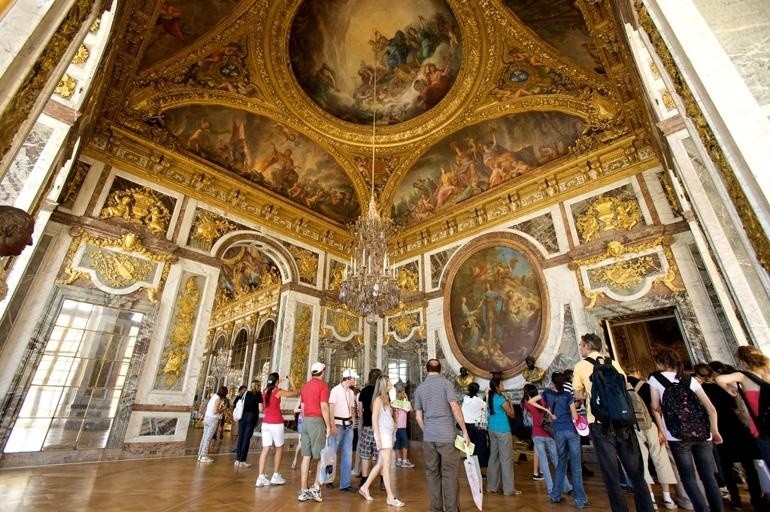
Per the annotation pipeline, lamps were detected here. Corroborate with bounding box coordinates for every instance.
[337,28,401,317]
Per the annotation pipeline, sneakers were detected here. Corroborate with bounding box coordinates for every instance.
[308,484,323,502]
[197,456,201,461]
[297,490,313,501]
[653,500,658,511]
[239,462,251,467]
[401,461,415,468]
[255,476,271,486]
[395,459,403,466]
[664,498,678,509]
[539,471,545,478]
[533,475,544,481]
[271,475,287,485]
[582,500,593,508]
[200,456,214,463]
[234,460,239,466]
[552,496,566,504]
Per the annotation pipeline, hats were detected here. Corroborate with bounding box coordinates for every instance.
[342,368,360,379]
[572,415,591,437]
[311,362,325,375]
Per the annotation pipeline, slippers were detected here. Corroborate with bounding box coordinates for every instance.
[291,463,297,470]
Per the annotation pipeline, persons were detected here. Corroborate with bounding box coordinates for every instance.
[191,344,770,512]
[570,332,657,512]
[452,281,542,373]
[0,205,35,258]
[328,268,345,291]
[607,240,625,258]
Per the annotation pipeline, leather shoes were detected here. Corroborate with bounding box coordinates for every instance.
[387,497,405,507]
[340,485,357,494]
[325,483,335,488]
[359,487,374,501]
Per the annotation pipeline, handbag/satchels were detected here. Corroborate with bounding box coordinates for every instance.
[541,418,554,436]
[318,435,337,485]
[736,369,770,439]
[464,443,484,511]
[474,393,489,431]
[233,391,248,421]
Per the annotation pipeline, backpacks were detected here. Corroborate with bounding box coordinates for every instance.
[584,355,641,432]
[631,379,653,430]
[649,370,711,441]
